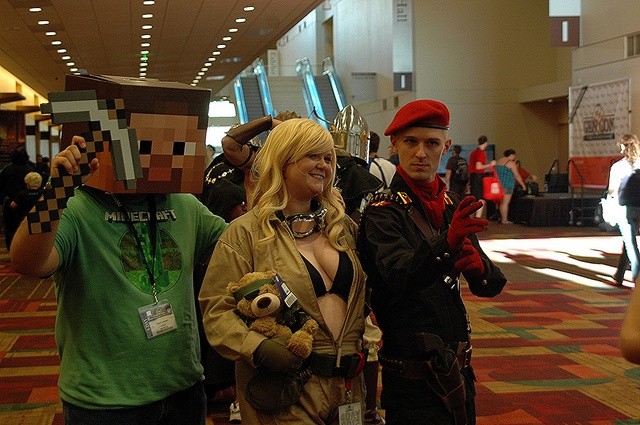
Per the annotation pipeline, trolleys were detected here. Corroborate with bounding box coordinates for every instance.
[567,159,598,227]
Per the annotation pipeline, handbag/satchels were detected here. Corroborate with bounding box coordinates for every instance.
[524,182,538,195]
[544,159,569,193]
[482,164,504,201]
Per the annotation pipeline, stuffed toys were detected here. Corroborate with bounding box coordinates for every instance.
[226,268,319,358]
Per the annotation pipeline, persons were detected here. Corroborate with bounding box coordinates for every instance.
[445,145,468,193]
[494,149,526,226]
[199,176,247,420]
[197,118,369,425]
[0,146,35,220]
[9,73,229,425]
[206,144,215,161]
[468,135,496,201]
[221,111,303,213]
[618,145,640,247]
[35,154,49,173]
[514,159,537,186]
[368,130,397,187]
[599,133,640,288]
[356,98,510,425]
[10,171,42,214]
[619,269,640,365]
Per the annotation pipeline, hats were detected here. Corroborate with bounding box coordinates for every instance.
[384,99,450,136]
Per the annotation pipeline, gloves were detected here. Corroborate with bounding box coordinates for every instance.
[454,238,484,276]
[254,339,302,395]
[447,196,489,258]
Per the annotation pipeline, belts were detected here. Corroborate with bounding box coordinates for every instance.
[302,349,369,378]
[375,346,473,376]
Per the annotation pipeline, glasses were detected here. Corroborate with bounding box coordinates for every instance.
[275,198,329,240]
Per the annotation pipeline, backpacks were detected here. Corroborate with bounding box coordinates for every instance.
[451,156,468,186]
